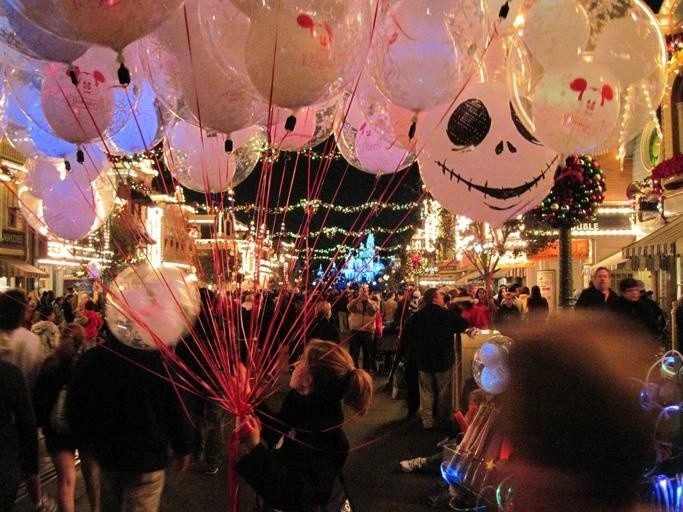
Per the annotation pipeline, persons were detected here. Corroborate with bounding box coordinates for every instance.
[2,266,683,511]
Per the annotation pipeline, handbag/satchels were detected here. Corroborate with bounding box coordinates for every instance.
[51,389,69,431]
[392,366,408,400]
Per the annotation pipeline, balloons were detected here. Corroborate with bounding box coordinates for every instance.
[1,2,667,349]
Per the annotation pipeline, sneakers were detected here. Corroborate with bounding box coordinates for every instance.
[450,497,485,511]
[399,457,422,472]
[427,493,449,507]
[32,498,57,512]
